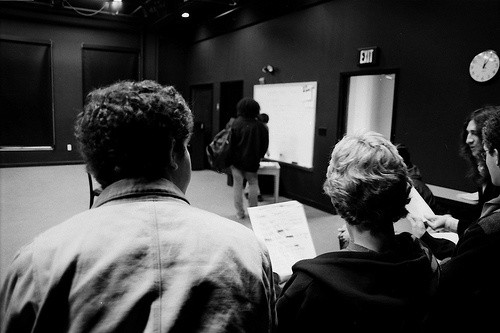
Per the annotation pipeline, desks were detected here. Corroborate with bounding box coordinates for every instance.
[256,161,280,202]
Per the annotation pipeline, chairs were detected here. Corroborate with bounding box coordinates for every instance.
[87,164,102,208]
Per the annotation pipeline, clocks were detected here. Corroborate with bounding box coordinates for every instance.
[469,50,500,83]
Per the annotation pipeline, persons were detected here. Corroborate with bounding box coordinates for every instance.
[0,80,276,333]
[273,131,440,333]
[226,97,268,218]
[397,105,500,333]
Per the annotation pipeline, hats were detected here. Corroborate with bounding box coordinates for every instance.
[238,97,260,118]
